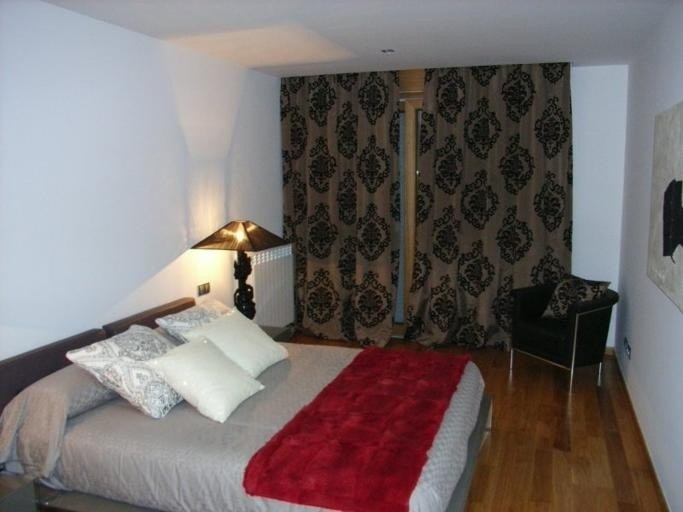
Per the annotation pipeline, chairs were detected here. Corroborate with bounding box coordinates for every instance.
[511,276,618,395]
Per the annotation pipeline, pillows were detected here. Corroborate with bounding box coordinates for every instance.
[146,336,266,425]
[65,324,183,420]
[183,306,290,379]
[155,296,233,341]
[539,273,611,320]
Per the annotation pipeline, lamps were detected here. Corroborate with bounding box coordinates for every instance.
[190,219,290,319]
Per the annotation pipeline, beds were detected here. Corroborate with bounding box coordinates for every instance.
[0,296,494,511]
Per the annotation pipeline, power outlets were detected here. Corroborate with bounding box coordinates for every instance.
[624,336,633,361]
